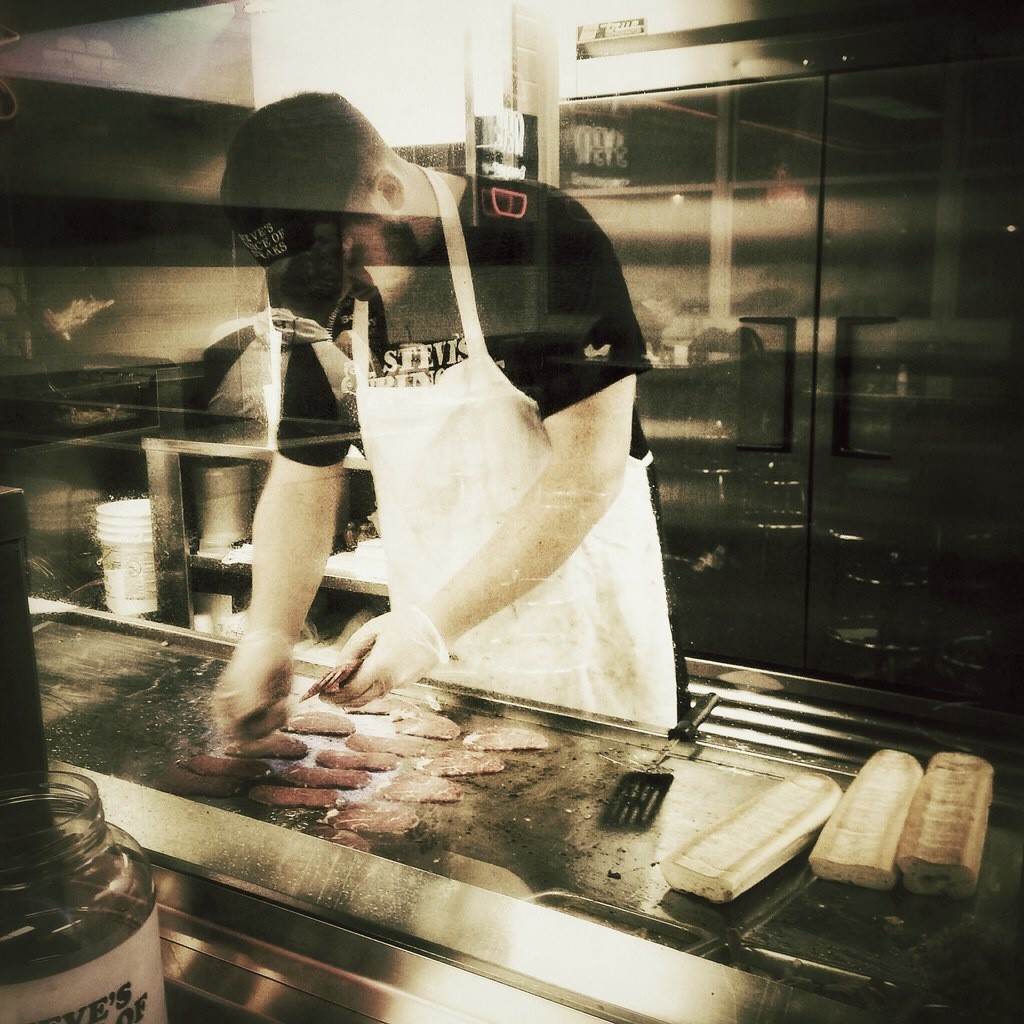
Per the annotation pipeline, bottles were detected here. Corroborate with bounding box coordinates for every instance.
[344,519,380,550]
[0,771,170,1024]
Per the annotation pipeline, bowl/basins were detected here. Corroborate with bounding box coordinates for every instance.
[825,514,1024,704]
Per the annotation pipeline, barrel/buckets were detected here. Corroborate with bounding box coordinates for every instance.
[95,498,159,616]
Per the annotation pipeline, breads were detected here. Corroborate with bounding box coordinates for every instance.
[896,752,994,900]
[660,771,844,904]
[809,748,923,890]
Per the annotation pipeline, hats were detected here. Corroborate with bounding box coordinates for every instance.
[219,168,352,323]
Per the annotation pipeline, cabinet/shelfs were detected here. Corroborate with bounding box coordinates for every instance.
[140,417,391,664]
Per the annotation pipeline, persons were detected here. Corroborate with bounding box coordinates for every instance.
[208,91,694,743]
[331,220,389,528]
